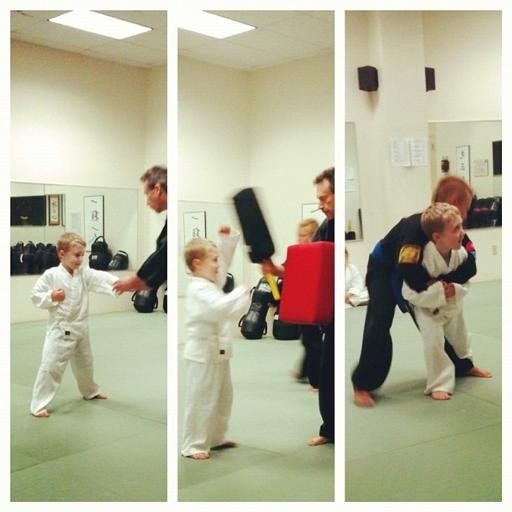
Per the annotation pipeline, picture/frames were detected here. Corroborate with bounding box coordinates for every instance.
[83,195,103,251]
[302,202,326,226]
[455,145,470,187]
[183,211,205,247]
[48,194,60,225]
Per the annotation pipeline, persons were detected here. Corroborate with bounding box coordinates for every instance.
[113,165,167,296]
[180,224,253,460]
[30,232,120,418]
[350,175,492,407]
[345,248,370,308]
[261,167,334,445]
[400,200,473,400]
[293,218,320,392]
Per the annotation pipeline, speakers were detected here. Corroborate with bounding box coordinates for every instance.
[358,65,378,92]
[425,66,436,91]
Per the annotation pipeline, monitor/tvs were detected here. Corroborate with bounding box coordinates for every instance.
[11,196,47,225]
[492,140,502,176]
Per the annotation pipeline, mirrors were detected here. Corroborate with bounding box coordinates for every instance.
[10,180,138,324]
[178,200,243,298]
[345,121,364,241]
[428,120,502,230]
